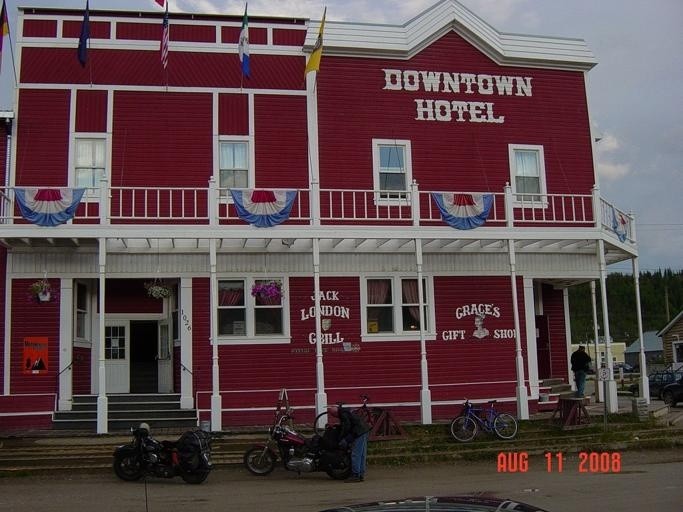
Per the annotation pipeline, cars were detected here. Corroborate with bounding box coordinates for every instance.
[628,367,683,406]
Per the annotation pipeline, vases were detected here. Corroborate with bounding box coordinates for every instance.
[38,291,51,301]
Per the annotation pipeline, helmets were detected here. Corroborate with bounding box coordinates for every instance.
[139,423,150,433]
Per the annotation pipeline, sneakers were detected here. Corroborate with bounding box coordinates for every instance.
[344,476,365,483]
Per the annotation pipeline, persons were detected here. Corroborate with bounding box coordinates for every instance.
[570,344,591,398]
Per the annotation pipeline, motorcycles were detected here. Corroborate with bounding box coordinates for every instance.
[243,407,356,480]
[111,423,214,485]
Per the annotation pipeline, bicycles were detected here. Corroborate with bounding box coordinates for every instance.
[450,396,518,441]
[313,392,386,439]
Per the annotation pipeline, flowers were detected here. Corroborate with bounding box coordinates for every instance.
[26,278,60,302]
[144,278,173,298]
[251,279,282,304]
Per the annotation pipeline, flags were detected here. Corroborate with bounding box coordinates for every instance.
[238,2,251,80]
[0,0,10,74]
[77,0,91,67]
[159,2,170,71]
[303,5,328,78]
